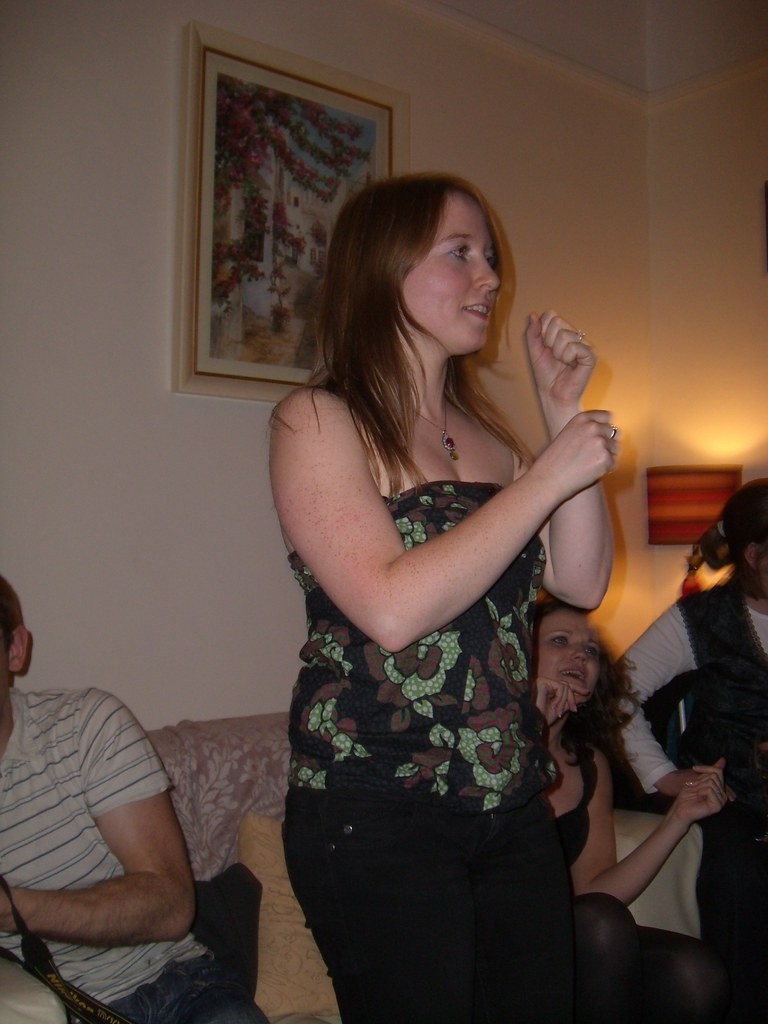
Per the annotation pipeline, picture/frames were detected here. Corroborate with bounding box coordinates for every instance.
[173,20,410,402]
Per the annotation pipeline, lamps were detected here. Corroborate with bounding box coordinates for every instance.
[647,464,741,597]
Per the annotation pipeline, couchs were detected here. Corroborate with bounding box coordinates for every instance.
[0,711,706,1024]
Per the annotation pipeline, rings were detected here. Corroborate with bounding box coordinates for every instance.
[713,787,720,793]
[575,329,586,342]
[610,422,617,440]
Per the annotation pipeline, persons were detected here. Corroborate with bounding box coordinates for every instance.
[1,578,272,1023]
[266,168,621,1024]
[534,600,726,1024]
[617,479,768,1024]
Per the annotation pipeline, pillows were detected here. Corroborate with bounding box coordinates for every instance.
[237,813,340,1018]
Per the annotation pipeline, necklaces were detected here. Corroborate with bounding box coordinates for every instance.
[408,402,459,459]
